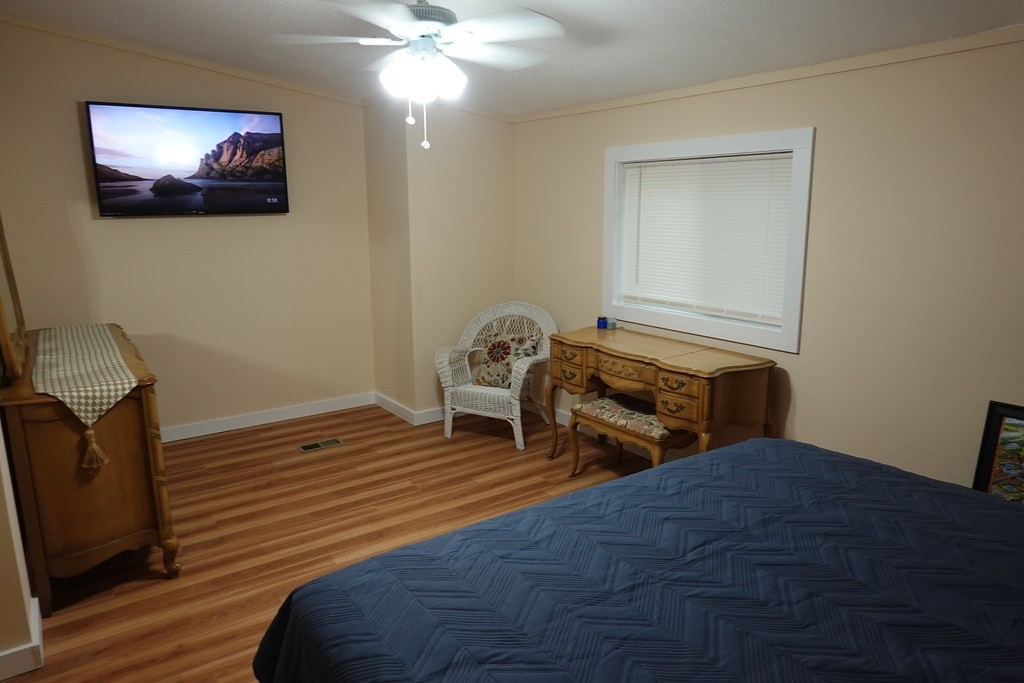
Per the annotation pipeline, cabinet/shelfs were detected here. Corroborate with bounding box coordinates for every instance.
[0,323,179,618]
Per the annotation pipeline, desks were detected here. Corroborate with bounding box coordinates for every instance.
[544,325,778,458]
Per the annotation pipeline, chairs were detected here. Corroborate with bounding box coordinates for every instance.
[434,301,561,451]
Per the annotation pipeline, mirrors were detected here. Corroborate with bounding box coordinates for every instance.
[1,212,29,379]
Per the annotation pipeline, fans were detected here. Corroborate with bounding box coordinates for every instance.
[265,0,568,73]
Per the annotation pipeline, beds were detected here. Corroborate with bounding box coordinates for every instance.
[271,437,1023,683]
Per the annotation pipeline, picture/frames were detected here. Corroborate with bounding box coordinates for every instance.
[972,399,1024,502]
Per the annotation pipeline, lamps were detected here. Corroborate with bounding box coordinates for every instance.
[378,36,471,149]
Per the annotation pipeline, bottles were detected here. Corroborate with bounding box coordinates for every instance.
[598,316,617,329]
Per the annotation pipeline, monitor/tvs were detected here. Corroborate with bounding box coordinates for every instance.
[84,99,290,217]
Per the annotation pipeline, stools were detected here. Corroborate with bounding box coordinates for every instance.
[568,392,698,479]
